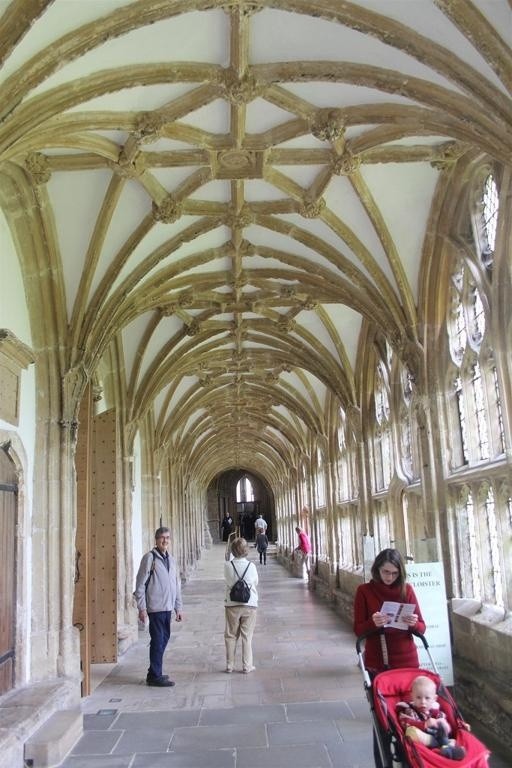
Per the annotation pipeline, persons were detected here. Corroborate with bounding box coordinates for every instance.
[397,676,472,760]
[135,527,184,687]
[220,512,232,541]
[254,528,268,565]
[223,537,259,674]
[254,515,267,540]
[294,527,311,579]
[352,548,427,768]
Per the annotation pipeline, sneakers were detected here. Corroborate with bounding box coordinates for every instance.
[162,675,169,680]
[243,666,255,674]
[226,666,236,673]
[439,745,466,761]
[147,679,175,687]
[433,721,448,746]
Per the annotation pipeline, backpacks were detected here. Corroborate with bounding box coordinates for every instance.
[230,561,252,603]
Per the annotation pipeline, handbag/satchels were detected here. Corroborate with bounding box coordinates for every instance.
[133,577,151,595]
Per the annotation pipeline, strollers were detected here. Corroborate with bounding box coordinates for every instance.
[356,625,490,768]
